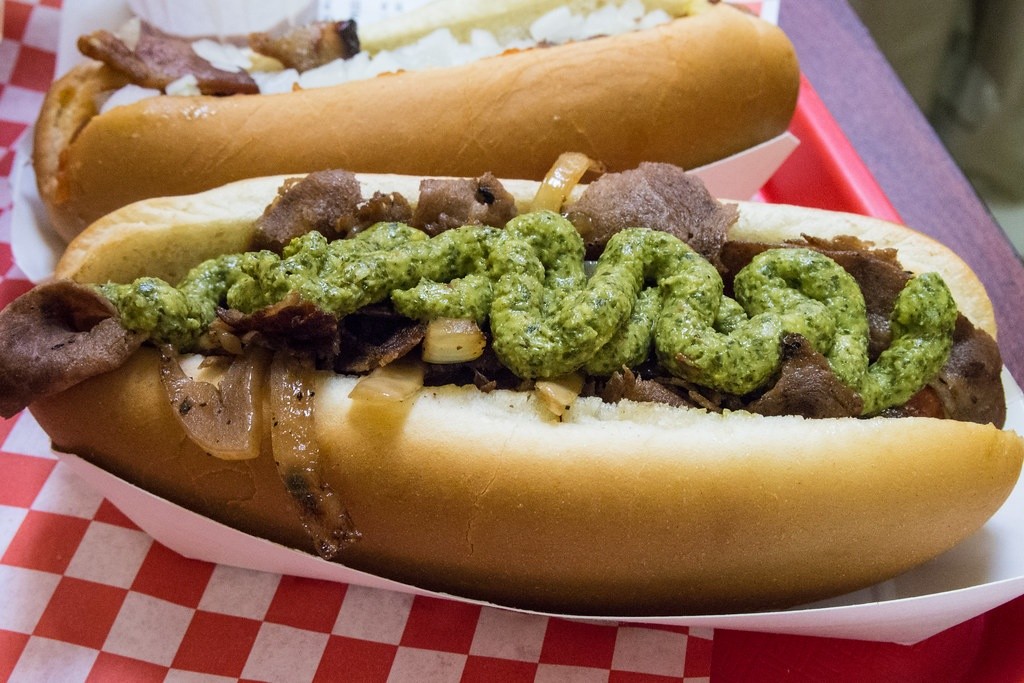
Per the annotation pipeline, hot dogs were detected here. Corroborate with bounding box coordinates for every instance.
[0,150,1022,619]
[32,0,800,243]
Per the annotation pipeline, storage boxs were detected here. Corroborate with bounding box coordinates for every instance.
[47,366,1024,645]
